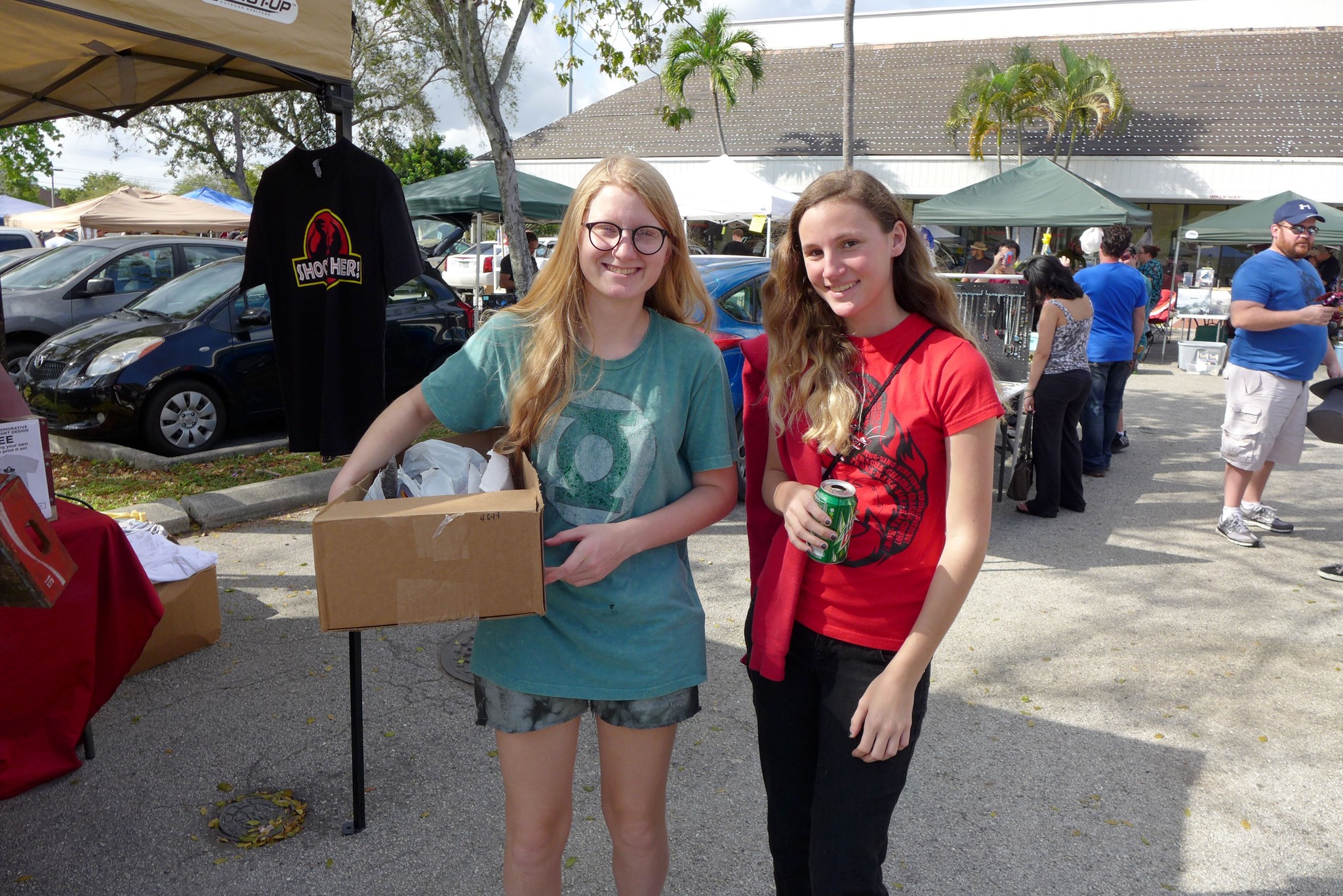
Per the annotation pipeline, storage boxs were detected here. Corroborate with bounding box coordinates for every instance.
[131,532,223,677]
[1186,362,1221,376]
[1177,340,1227,370]
[312,427,546,633]
[0,414,78,607]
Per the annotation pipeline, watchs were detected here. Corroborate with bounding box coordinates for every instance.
[1024,387,1034,393]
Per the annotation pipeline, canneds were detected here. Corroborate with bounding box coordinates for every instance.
[805,479,858,563]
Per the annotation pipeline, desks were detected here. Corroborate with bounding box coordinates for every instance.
[0,498,165,798]
[995,380,1029,502]
[1177,314,1230,367]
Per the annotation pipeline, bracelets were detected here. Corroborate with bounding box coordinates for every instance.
[1024,395,1034,400]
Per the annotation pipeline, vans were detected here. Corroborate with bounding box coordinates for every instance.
[0,228,42,250]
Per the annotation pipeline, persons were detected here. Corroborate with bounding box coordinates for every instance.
[45,160,1343,896]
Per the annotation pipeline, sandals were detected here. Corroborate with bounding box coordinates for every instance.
[1017,500,1057,518]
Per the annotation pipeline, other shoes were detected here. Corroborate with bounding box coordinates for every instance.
[1091,465,1111,476]
[1110,430,1129,453]
[1317,563,1343,582]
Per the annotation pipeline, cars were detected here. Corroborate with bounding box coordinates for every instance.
[644,253,774,502]
[0,235,475,458]
[425,237,565,294]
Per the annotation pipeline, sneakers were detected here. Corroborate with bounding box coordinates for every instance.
[1240,503,1294,532]
[1215,513,1259,546]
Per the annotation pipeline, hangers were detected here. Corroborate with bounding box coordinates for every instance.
[296,80,343,146]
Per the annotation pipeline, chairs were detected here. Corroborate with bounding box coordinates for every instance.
[1148,289,1177,343]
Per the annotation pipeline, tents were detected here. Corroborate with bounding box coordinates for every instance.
[0,0,365,837]
[1,156,1342,365]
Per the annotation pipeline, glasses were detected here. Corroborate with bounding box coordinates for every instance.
[1312,247,1321,251]
[581,222,670,256]
[1139,251,1147,254]
[1120,254,1135,260]
[1277,224,1320,235]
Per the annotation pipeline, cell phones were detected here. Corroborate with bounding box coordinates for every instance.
[1323,293,1339,307]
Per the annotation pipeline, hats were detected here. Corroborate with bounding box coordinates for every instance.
[970,242,988,251]
[1273,199,1326,225]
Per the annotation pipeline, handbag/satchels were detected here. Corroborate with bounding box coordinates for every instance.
[1007,411,1034,501]
